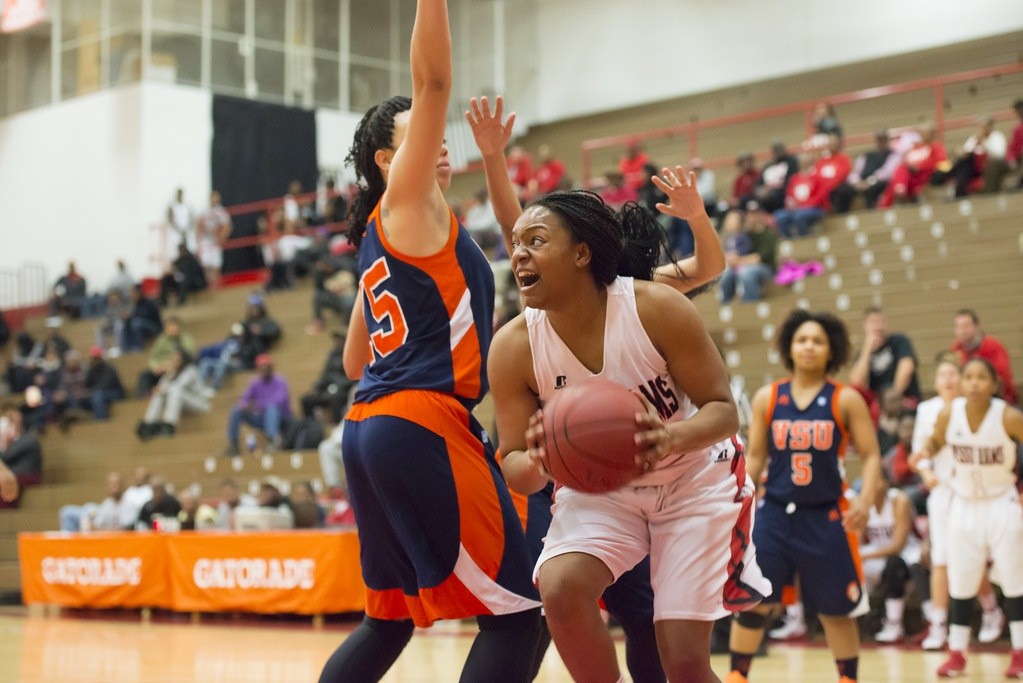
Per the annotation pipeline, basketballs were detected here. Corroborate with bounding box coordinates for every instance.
[535,376,653,494]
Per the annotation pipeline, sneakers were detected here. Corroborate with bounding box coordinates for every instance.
[978,606,1004,644]
[876,617,906,642]
[769,616,807,641]
[922,623,946,649]
[1008,650,1023,678]
[938,649,965,677]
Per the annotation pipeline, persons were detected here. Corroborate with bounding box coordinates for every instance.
[719,202,778,303]
[321,0,542,682]
[465,94,724,683]
[858,468,919,642]
[58,468,353,533]
[466,190,508,260]
[722,309,885,682]
[769,578,807,638]
[876,398,918,488]
[909,359,1004,648]
[907,359,1023,678]
[731,99,1023,237]
[946,309,1016,406]
[603,139,714,257]
[486,191,771,683]
[507,144,571,204]
[1,179,358,503]
[849,308,921,397]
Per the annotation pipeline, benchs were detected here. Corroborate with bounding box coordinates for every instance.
[1,28,1023,606]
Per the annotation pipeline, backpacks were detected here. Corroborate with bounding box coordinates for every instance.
[283,418,322,450]
